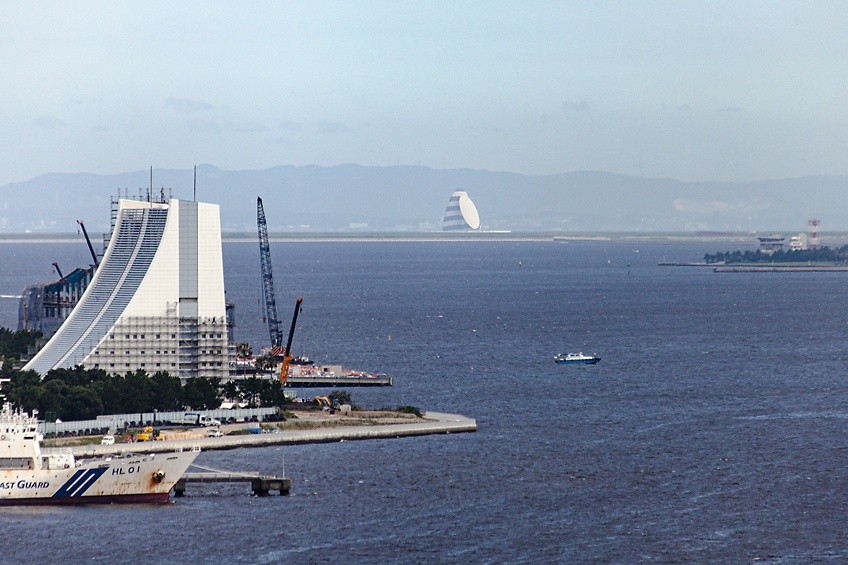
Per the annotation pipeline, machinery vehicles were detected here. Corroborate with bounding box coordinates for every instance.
[136,426,167,442]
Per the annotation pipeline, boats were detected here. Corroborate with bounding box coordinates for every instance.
[0,378,202,504]
[554,352,603,364]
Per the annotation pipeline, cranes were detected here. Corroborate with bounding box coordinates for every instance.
[257,196,287,363]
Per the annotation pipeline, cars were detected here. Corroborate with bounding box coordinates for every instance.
[101,436,115,446]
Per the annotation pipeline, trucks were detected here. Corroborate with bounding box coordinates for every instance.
[199,415,221,426]
[207,429,223,438]
